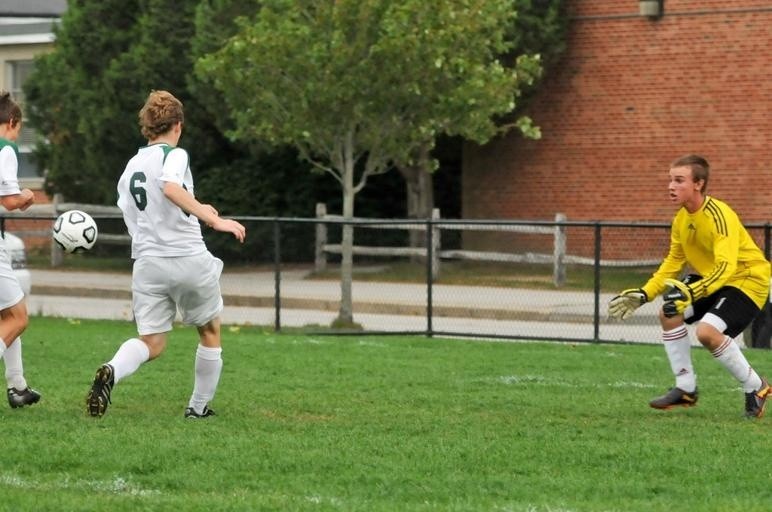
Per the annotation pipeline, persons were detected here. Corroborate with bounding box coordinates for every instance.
[605,150,772,422]
[0,88,42,409]
[82,88,248,422]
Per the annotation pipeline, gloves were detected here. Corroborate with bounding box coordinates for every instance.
[663,278,693,319]
[607,289,647,319]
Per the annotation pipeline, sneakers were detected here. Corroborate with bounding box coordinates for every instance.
[744,378,772,417]
[7,384,42,409]
[184,406,218,419]
[86,362,114,417]
[650,387,698,409]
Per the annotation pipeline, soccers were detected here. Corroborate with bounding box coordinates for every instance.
[53,210,97,255]
